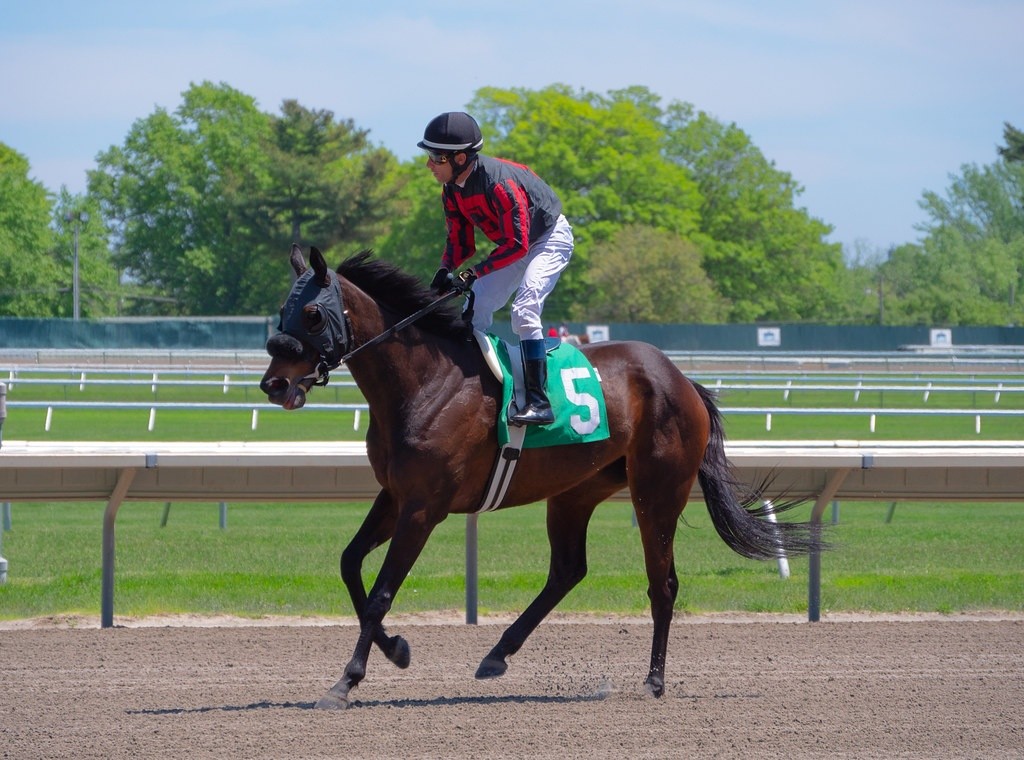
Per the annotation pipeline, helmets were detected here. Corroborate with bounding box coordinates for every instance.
[416,111,484,154]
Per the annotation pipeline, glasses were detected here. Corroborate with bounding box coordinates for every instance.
[425,149,460,165]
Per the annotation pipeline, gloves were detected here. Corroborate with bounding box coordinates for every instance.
[453,272,475,294]
[430,268,450,289]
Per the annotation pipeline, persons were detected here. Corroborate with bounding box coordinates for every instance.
[557,321,568,338]
[415,112,574,429]
[546,324,557,337]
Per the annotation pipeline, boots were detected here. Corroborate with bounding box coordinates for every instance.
[510,339,555,425]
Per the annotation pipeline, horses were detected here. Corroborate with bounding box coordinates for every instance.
[260,244,843,710]
